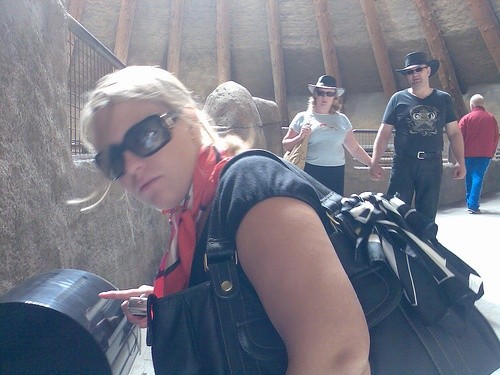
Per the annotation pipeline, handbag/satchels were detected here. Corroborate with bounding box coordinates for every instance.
[284,116,310,170]
[146,148,500,375]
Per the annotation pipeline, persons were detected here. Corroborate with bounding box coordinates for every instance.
[370,51,466,221]
[282,75,383,196]
[459,94,500,213]
[79,66,500,375]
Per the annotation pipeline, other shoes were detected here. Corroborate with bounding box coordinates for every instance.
[466,208,480,214]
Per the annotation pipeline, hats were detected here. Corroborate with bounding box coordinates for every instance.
[307,74,345,97]
[396,51,440,77]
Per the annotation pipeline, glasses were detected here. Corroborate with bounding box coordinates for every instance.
[95,111,177,182]
[407,67,426,74]
[317,89,334,97]
